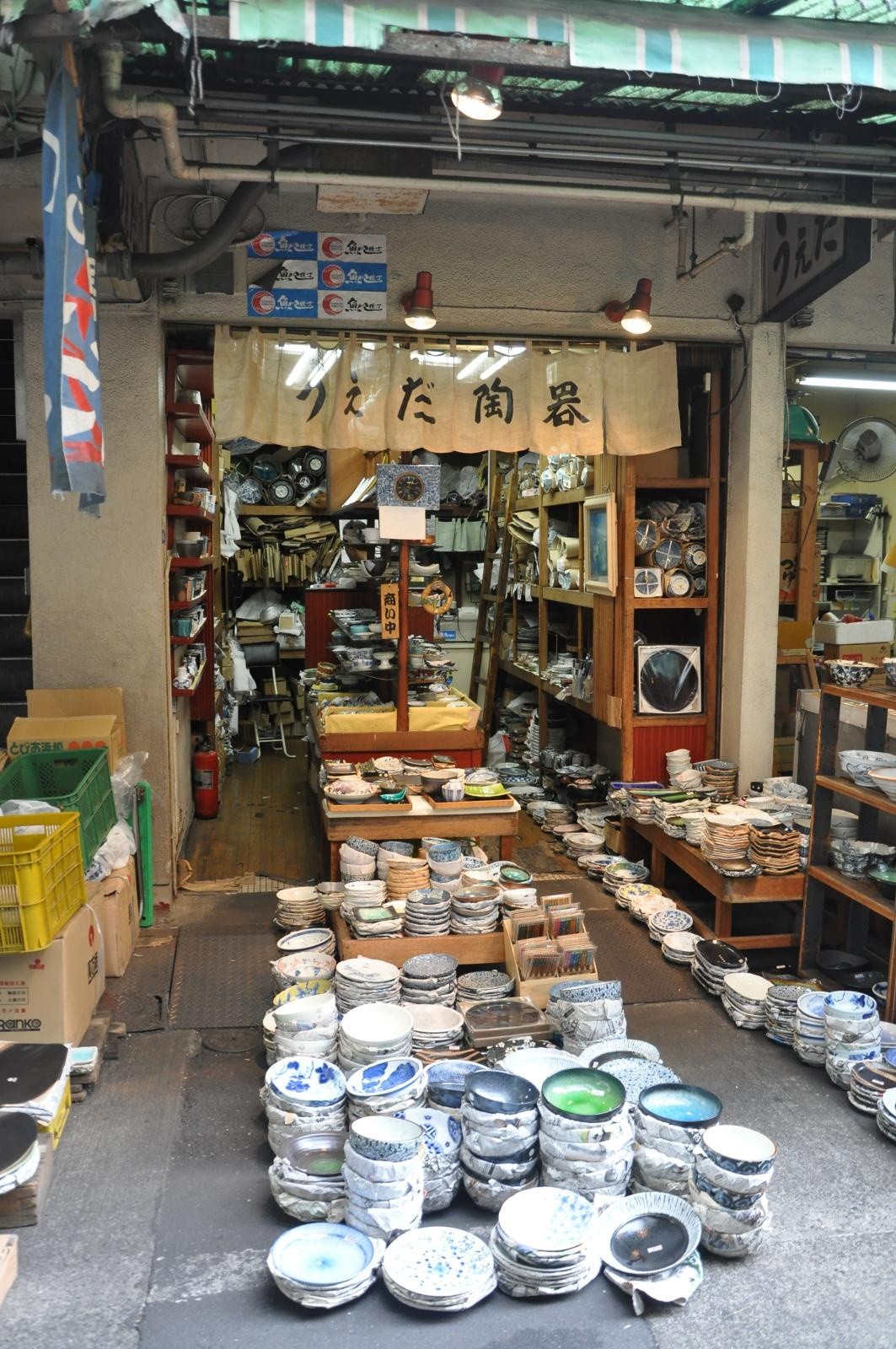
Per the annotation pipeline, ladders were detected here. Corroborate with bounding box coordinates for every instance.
[469,472,518,767]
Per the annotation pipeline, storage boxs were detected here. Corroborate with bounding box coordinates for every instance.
[0,688,152,1049]
[785,505,894,687]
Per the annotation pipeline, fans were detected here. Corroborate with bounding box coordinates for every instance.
[826,416,896,486]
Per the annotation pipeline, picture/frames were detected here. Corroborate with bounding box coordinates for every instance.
[583,492,617,597]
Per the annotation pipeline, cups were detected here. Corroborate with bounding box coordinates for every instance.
[174,442,207,685]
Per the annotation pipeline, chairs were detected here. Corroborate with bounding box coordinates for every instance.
[240,641,297,761]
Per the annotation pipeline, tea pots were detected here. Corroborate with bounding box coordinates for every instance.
[540,746,613,798]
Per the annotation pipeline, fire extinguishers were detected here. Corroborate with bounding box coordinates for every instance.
[194,734,219,820]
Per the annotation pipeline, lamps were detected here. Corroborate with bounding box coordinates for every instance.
[604,278,653,335]
[402,271,436,330]
[789,364,896,390]
[450,35,510,121]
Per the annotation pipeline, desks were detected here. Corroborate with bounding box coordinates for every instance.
[619,814,805,952]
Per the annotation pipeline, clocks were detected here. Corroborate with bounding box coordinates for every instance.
[377,464,440,511]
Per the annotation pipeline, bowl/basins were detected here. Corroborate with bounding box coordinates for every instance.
[666,748,858,871]
[837,749,896,803]
[420,771,459,793]
[441,780,465,801]
[341,832,532,890]
[332,608,396,670]
[863,863,896,899]
[342,1113,427,1242]
[461,1070,775,1256]
[546,980,629,1053]
[824,658,896,689]
[360,528,388,576]
[795,989,881,1088]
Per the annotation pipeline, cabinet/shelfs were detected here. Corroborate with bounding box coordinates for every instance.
[306,585,523,974]
[165,319,216,723]
[494,371,719,817]
[770,436,837,777]
[794,680,896,1022]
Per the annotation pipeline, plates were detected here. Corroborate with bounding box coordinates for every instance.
[263,882,537,1058]
[607,781,801,875]
[265,1041,678,1215]
[566,833,707,960]
[816,951,889,1000]
[407,684,468,707]
[849,1021,896,1141]
[267,1187,704,1312]
[496,760,582,835]
[323,754,507,804]
[829,840,896,878]
[517,627,574,684]
[693,938,813,1044]
